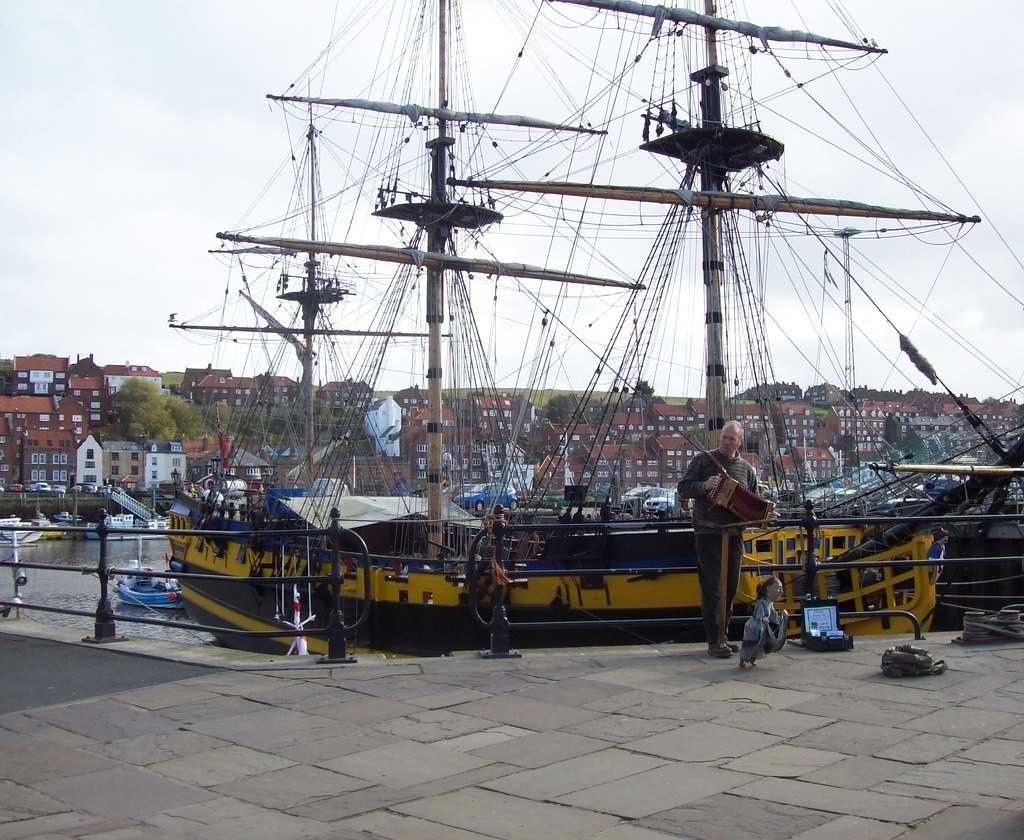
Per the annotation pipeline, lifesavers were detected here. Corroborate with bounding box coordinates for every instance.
[204,479,216,491]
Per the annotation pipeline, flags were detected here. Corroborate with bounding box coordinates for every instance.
[216,416,232,469]
[165,552,170,564]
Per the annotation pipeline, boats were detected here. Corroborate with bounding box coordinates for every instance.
[116,558,183,609]
[0,505,148,548]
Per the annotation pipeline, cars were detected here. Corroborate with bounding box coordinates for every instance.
[642,489,680,517]
[0,480,125,497]
[609,486,667,516]
[454,482,517,513]
[443,483,478,503]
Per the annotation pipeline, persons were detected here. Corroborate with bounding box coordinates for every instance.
[739,576,788,670]
[928,528,949,583]
[678,420,781,658]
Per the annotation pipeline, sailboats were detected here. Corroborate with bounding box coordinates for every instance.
[150,0,1024,663]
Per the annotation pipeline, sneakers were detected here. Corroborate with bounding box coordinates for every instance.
[725,641,739,653]
[708,641,733,657]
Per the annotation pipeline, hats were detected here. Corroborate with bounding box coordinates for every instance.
[933,527,949,542]
